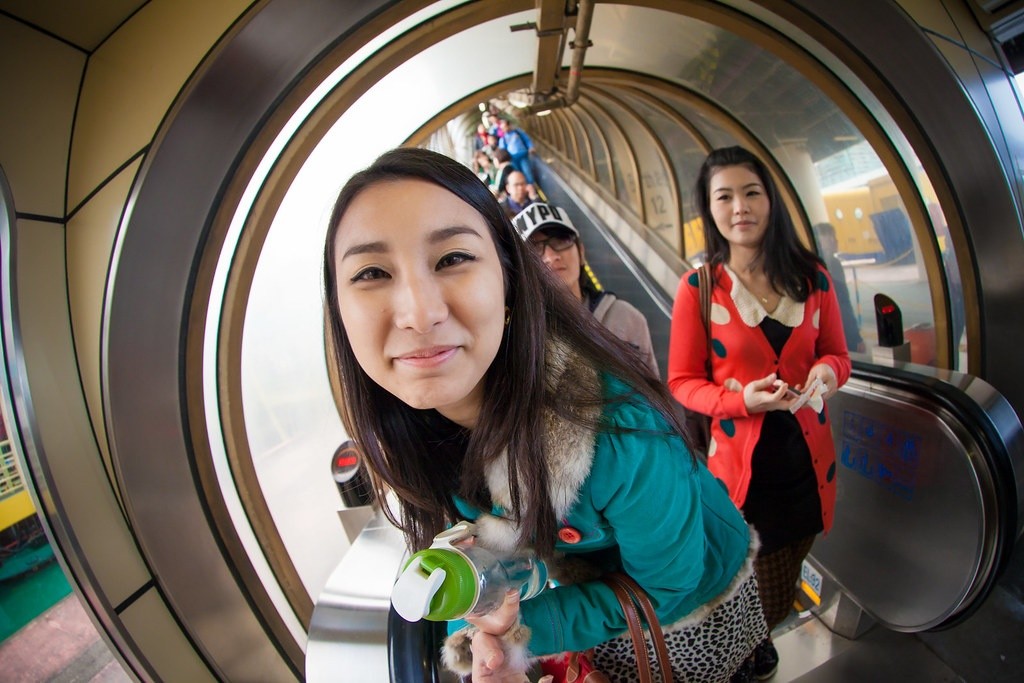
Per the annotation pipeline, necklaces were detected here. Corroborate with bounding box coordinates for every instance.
[749,284,773,304]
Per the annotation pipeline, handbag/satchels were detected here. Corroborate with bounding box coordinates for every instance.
[538,572,675,683]
[683,411,711,460]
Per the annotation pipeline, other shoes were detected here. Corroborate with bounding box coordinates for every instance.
[753,637,778,680]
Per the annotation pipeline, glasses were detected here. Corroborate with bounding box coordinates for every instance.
[533,230,576,257]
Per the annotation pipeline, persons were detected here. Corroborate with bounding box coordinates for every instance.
[506,202,663,380]
[665,144,854,635]
[811,223,866,352]
[473,110,539,217]
[326,148,778,683]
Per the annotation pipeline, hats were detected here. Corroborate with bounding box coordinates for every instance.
[510,202,580,237]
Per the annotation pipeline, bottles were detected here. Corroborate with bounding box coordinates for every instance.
[390,525,549,622]
